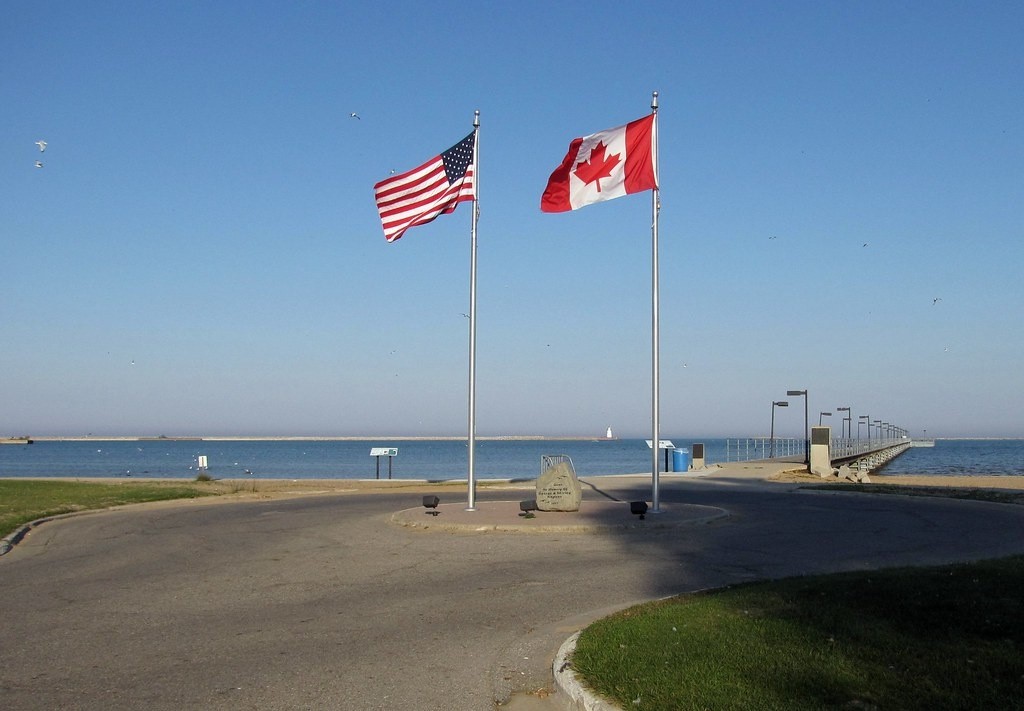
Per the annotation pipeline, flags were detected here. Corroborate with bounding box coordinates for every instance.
[373,128,474,244]
[540,112,658,213]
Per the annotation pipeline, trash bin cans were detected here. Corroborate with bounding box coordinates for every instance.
[672,447,689,472]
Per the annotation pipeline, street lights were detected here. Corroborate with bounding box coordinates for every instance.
[768,399,790,461]
[836,407,854,439]
[859,415,873,440]
[855,421,865,438]
[866,418,910,442]
[787,389,814,465]
[841,417,851,439]
[819,412,832,427]
